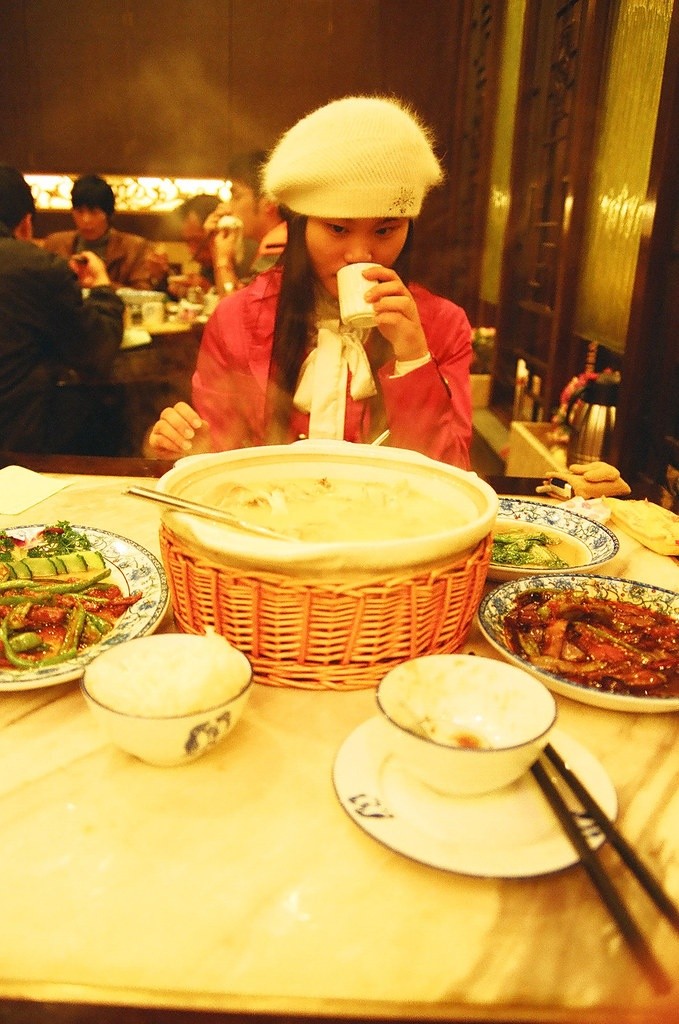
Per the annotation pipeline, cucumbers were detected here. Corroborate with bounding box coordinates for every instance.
[0,551,107,583]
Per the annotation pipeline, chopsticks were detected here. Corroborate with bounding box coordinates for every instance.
[471,650,679,997]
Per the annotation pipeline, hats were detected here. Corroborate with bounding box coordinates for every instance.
[265,96,443,219]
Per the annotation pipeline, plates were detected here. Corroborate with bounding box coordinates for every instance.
[0,525,170,692]
[487,497,619,581]
[332,716,616,878]
[478,575,678,714]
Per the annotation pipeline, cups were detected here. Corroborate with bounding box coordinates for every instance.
[336,262,386,328]
[142,303,164,326]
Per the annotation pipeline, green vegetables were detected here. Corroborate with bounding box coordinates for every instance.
[491,530,571,569]
[0,520,93,562]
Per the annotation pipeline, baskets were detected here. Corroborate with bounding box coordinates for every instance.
[157,521,493,691]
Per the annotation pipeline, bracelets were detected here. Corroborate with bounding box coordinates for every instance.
[212,265,235,271]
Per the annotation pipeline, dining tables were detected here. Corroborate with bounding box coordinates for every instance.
[0,459,679,1024]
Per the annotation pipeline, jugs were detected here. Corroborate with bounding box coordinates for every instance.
[561,379,619,469]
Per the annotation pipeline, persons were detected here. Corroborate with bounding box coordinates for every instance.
[0,165,134,457]
[172,147,290,296]
[141,95,476,473]
[34,175,168,296]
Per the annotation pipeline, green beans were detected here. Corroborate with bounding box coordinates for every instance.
[0,567,117,668]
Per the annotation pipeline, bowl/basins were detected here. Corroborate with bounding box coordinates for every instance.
[375,654,556,794]
[155,445,498,569]
[82,633,251,767]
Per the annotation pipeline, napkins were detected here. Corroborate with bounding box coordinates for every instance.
[0,465,71,515]
